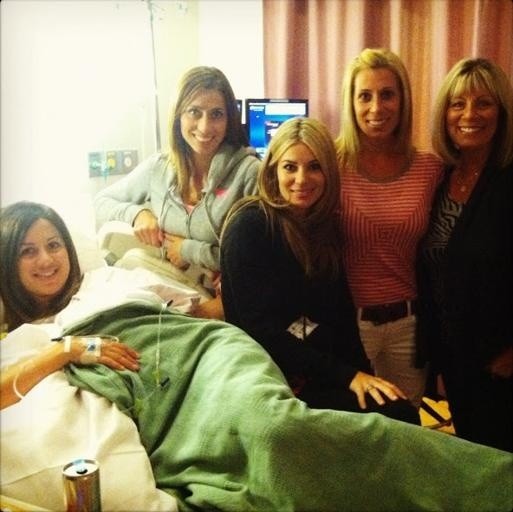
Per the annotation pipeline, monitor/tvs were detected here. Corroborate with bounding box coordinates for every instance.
[246,99,309,153]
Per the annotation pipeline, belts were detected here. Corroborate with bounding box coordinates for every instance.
[360,300,417,326]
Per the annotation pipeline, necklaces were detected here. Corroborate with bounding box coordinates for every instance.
[454,171,479,193]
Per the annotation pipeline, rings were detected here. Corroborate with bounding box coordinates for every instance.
[367,385,374,391]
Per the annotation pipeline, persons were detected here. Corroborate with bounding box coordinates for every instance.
[219,116,424,428]
[333,46,443,414]
[1,199,223,512]
[93,65,263,296]
[417,58,513,453]
